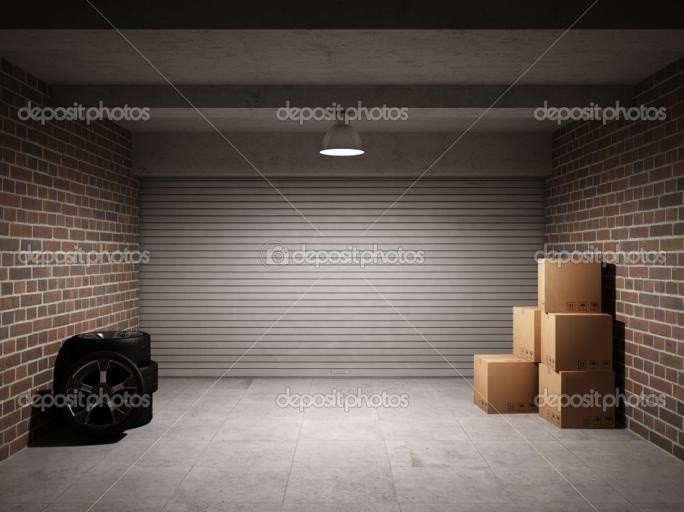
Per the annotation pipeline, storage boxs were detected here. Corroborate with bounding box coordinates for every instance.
[472,257,623,427]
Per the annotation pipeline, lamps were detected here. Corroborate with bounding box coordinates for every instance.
[319,112,366,156]
